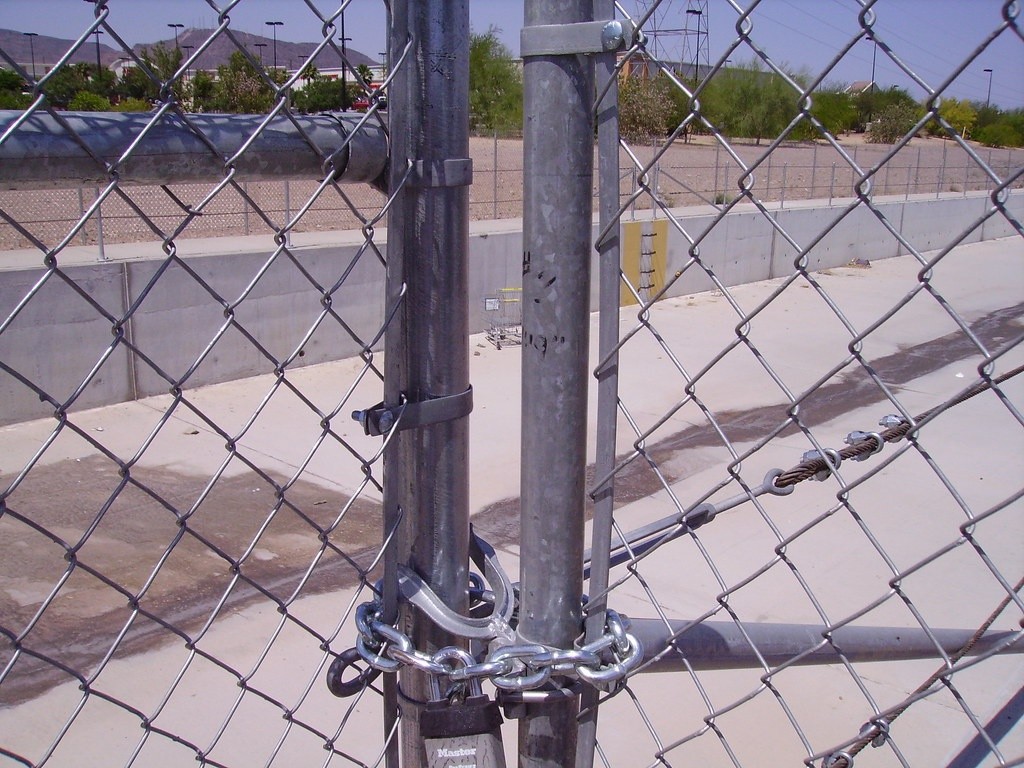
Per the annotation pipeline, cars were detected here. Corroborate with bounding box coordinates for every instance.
[351,90,387,110]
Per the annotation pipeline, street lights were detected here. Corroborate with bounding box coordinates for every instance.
[865,36,878,122]
[684,8,703,94]
[180,45,195,81]
[166,23,185,48]
[253,43,268,67]
[984,69,994,109]
[118,57,129,78]
[298,55,310,86]
[378,53,386,83]
[21,32,38,78]
[122,48,135,69]
[338,38,352,82]
[90,29,105,83]
[265,21,284,84]
